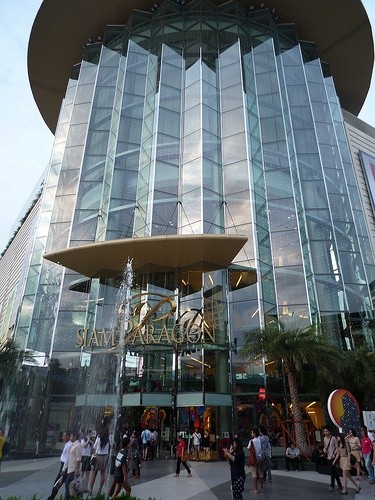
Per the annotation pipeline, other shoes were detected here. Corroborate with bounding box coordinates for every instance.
[249,490,257,494]
[259,488,264,492]
[133,476,137,479]
[356,487,361,493]
[85,489,89,493]
[174,474,179,477]
[328,487,334,491]
[186,474,192,477]
[340,491,348,495]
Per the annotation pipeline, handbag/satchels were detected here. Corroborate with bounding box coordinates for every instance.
[325,453,327,463]
[199,438,202,443]
[69,478,81,495]
[350,454,357,465]
[182,453,189,461]
[90,454,96,466]
[248,440,256,466]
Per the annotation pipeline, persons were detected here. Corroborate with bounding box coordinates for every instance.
[141,366,147,392]
[48,427,140,500]
[141,426,158,460]
[173,434,193,477]
[229,425,272,493]
[322,426,375,494]
[191,427,211,463]
[222,438,246,500]
[285,442,301,471]
